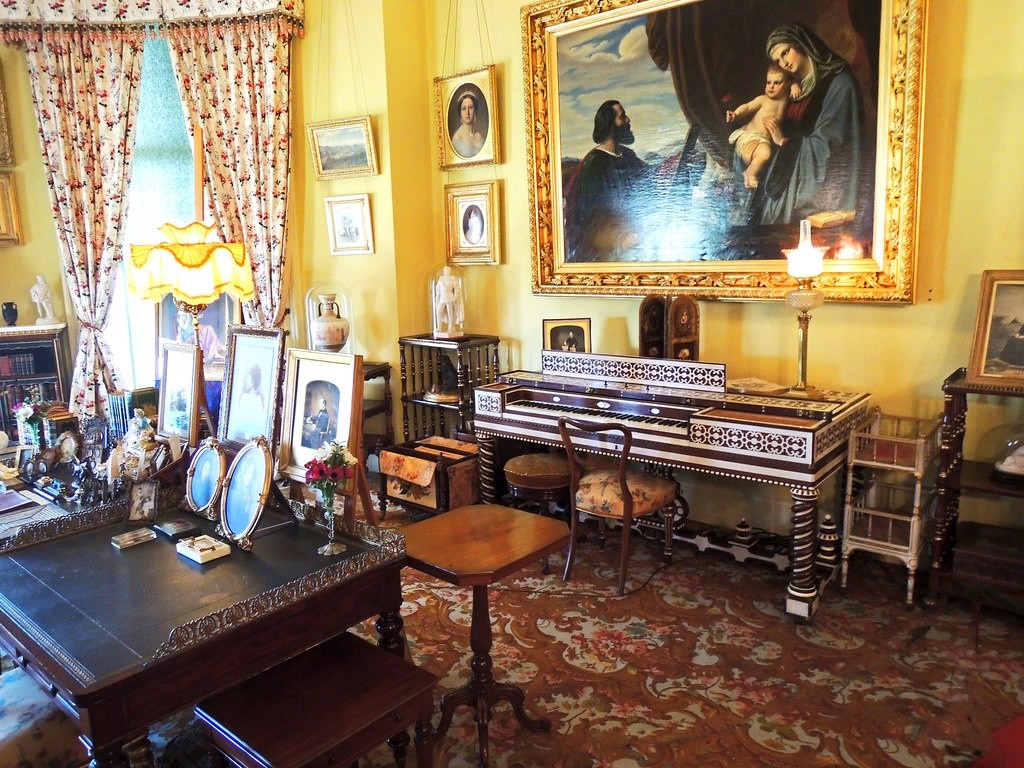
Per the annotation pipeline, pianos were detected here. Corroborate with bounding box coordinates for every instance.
[473,368,872,624]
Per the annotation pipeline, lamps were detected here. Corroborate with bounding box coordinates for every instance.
[125,216,257,436]
[781,219,831,392]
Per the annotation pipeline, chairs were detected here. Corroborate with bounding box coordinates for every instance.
[554,413,685,596]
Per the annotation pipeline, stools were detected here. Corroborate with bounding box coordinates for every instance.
[394,502,574,768]
[502,451,585,576]
[0,664,156,768]
[193,629,441,768]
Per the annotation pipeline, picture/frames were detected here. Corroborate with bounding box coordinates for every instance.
[542,317,592,354]
[442,179,503,268]
[304,112,381,184]
[519,0,933,307]
[156,335,204,448]
[14,444,41,474]
[276,347,364,499]
[212,433,275,551]
[964,269,1024,390]
[0,169,26,248]
[123,477,162,527]
[432,64,504,173]
[0,57,18,168]
[216,322,286,464]
[324,192,375,257]
[178,435,227,522]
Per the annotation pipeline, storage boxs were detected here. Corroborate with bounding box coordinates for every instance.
[376,433,482,516]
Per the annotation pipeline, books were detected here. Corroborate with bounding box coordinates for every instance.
[109,387,158,442]
[0,351,80,448]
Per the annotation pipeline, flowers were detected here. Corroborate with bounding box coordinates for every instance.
[9,384,53,444]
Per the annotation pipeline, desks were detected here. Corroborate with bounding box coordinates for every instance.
[471,369,884,629]
[0,432,410,768]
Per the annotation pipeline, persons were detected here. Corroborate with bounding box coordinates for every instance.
[193,457,215,508]
[562,331,577,352]
[29,272,56,323]
[434,266,462,336]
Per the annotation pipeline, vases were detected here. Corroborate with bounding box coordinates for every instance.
[309,290,351,354]
[24,421,41,454]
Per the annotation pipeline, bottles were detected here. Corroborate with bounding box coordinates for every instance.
[311,294,350,354]
[1,301,18,327]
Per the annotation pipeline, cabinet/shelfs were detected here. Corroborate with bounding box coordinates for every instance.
[918,365,1024,609]
[362,359,395,500]
[397,332,501,442]
[841,404,946,608]
[0,320,70,443]
